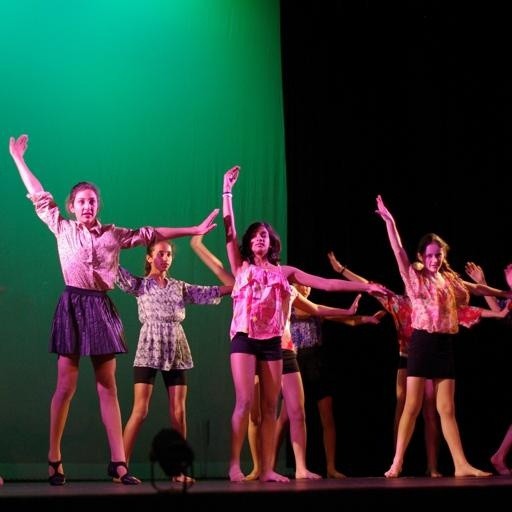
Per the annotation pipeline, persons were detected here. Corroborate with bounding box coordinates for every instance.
[113,239,235,483]
[222,164,387,483]
[7,133,221,487]
[328,249,512,481]
[184,235,364,478]
[464,257,512,477]
[372,194,494,478]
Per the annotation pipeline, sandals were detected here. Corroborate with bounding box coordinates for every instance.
[47,459,66,485]
[108,462,142,484]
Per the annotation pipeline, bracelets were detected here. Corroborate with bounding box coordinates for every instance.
[339,263,347,275]
[221,190,233,199]
